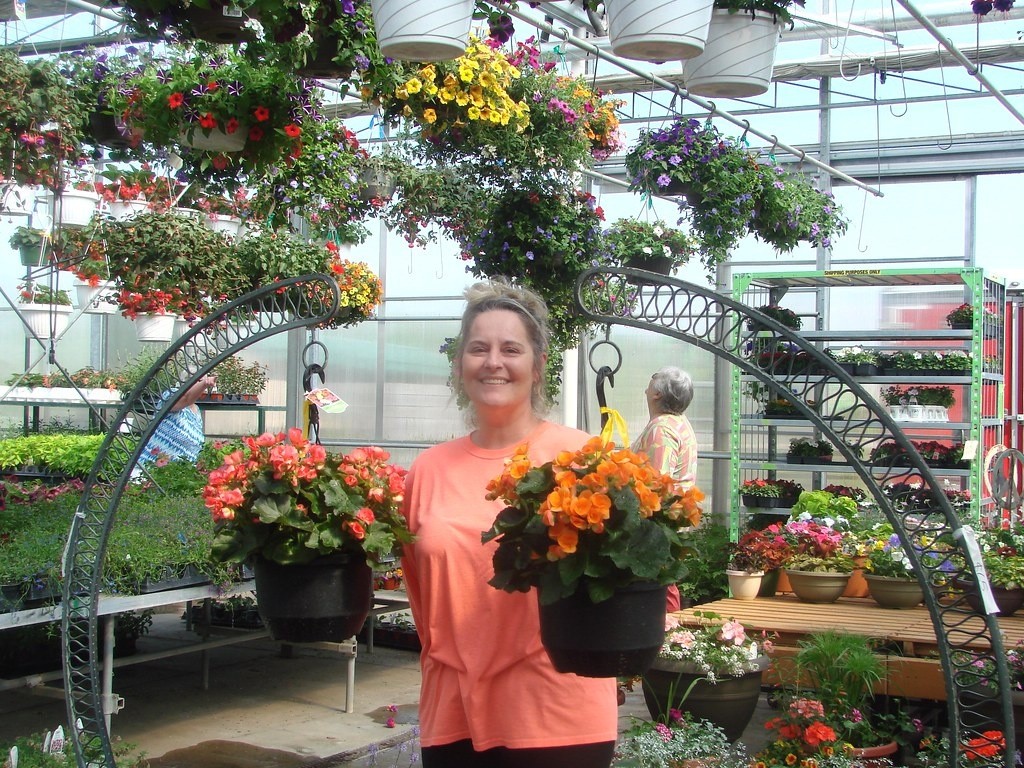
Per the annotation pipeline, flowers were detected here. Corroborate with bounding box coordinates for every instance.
[1,0,1021,766]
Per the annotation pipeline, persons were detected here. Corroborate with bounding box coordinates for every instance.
[399,275,619,768]
[126,364,219,484]
[628,365,697,494]
